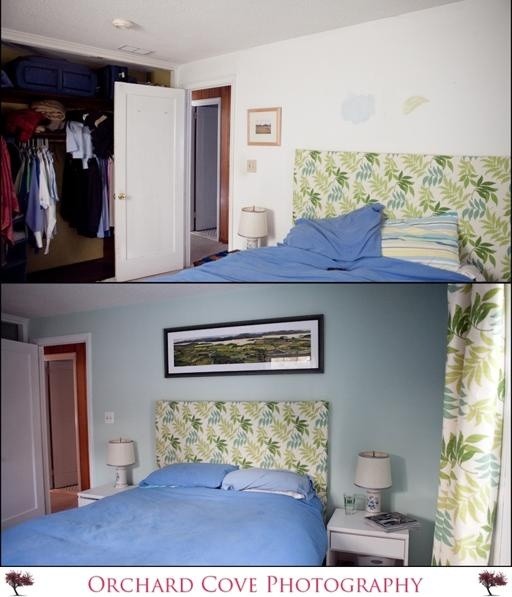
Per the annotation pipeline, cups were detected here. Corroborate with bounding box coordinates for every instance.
[355,493,366,509]
[342,492,356,513]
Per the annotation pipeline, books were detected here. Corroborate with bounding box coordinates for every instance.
[365,511,420,532]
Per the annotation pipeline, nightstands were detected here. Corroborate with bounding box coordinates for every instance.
[325,508,409,566]
[77,483,136,509]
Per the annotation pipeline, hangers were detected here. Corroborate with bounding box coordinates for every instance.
[19,138,54,164]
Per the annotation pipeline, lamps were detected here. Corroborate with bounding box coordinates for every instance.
[237,205,268,250]
[353,451,392,513]
[106,436,135,488]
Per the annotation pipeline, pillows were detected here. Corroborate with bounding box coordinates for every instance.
[221,468,314,499]
[139,463,239,490]
[382,212,460,267]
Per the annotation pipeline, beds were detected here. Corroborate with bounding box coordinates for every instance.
[132,150,511,283]
[1,399,329,566]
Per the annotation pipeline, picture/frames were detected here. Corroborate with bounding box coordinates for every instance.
[163,313,324,377]
[247,106,282,145]
